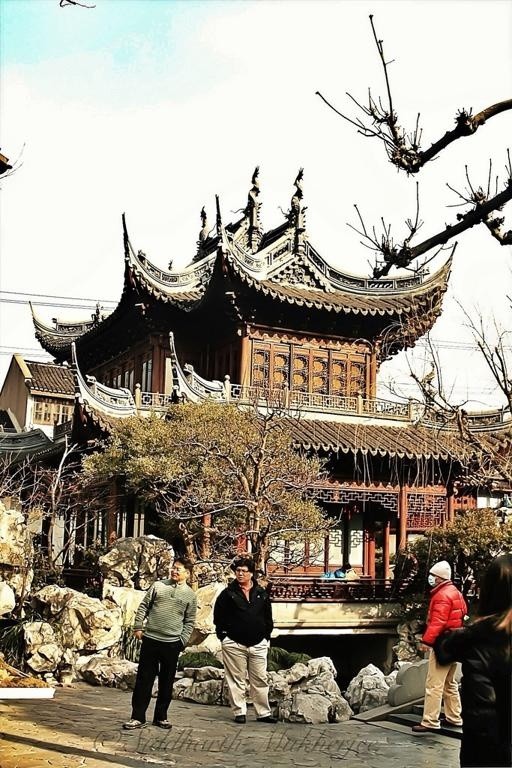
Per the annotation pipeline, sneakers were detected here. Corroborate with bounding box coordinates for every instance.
[122,718,148,730]
[439,718,463,728]
[256,714,279,723]
[234,715,246,723]
[153,719,173,729]
[411,724,441,733]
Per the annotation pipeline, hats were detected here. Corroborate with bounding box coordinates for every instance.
[428,560,452,580]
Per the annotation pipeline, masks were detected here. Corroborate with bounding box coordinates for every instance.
[428,574,437,587]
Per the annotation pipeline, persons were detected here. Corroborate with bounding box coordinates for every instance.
[412,560,468,731]
[122,556,197,730]
[335,561,360,592]
[434,554,512,765]
[212,559,278,724]
